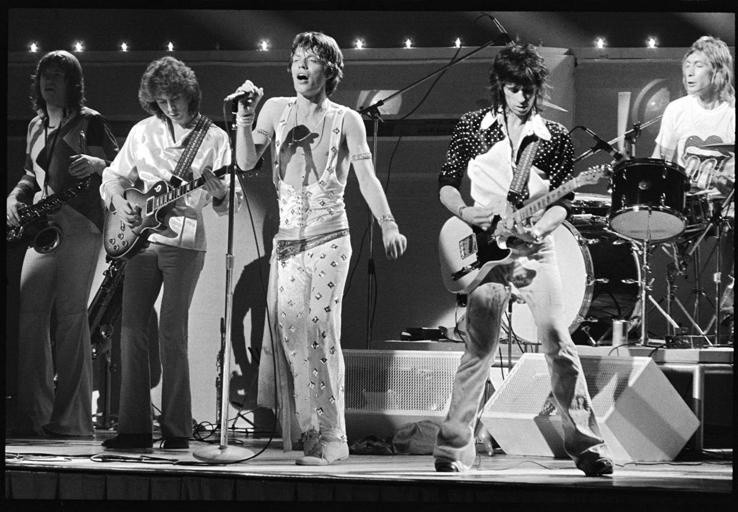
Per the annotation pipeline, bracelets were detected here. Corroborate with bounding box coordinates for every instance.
[458,205,465,215]
[236,113,254,126]
[378,214,395,227]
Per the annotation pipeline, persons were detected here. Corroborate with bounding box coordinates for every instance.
[235,31,408,466]
[5,49,118,436]
[434,40,614,480]
[98,55,243,449]
[650,37,737,347]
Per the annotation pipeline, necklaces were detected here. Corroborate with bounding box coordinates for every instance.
[287,100,331,150]
[47,125,57,128]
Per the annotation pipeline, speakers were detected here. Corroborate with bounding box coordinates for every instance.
[476,352,700,463]
[278,350,498,454]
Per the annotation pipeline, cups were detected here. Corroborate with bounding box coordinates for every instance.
[611,321,630,348]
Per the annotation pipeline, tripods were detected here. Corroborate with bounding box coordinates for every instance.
[597,175,735,347]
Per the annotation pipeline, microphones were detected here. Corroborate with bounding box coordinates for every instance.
[493,15,517,51]
[224,86,256,102]
[585,127,625,163]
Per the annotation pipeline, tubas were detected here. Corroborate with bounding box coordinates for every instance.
[4,132,96,253]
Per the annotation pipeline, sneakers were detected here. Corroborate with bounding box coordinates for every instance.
[102,434,153,451]
[164,437,191,453]
[295,438,349,466]
[434,456,469,472]
[302,430,321,455]
[576,450,613,477]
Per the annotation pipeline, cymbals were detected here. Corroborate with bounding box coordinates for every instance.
[706,144,736,154]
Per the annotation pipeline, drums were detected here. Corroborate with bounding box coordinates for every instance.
[686,188,714,232]
[502,215,642,345]
[573,193,615,227]
[609,158,689,242]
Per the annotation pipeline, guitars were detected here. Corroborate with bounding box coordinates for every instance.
[438,162,613,294]
[103,159,264,260]
